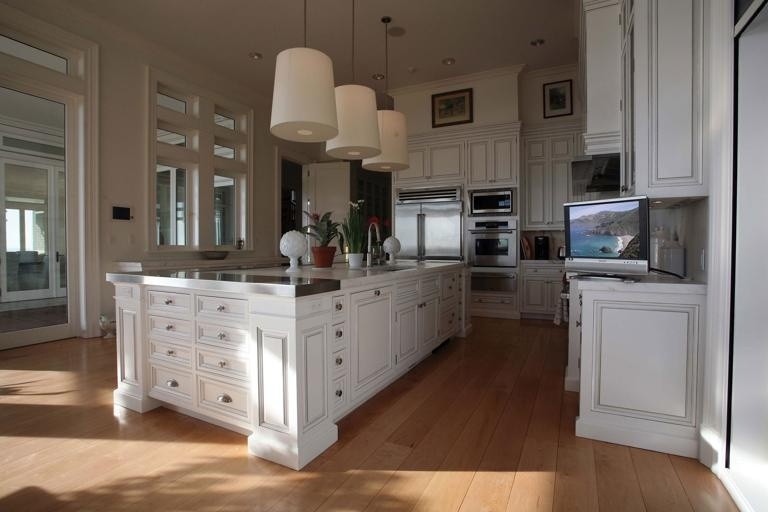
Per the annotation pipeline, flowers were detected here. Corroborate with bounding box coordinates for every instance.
[298,208,342,246]
[338,200,371,254]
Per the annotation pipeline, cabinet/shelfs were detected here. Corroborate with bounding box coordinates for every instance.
[618,0,708,208]
[393,120,585,320]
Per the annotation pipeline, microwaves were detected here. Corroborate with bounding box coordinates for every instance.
[469,187,515,214]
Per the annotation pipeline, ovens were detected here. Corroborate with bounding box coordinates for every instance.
[469,268,517,307]
[469,216,518,266]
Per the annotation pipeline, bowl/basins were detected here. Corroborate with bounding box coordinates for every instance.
[200,250,230,260]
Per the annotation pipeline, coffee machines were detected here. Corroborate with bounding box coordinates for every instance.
[534,236,549,260]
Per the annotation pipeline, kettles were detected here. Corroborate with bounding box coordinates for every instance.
[556,245,566,260]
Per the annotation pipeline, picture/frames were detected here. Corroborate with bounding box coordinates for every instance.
[542,78,574,119]
[430,88,474,128]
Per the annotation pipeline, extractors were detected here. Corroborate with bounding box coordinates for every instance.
[584,155,620,193]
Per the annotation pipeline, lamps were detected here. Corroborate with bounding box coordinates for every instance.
[268,0,339,143]
[280,230,308,273]
[382,236,401,264]
[326,1,382,160]
[359,16,411,173]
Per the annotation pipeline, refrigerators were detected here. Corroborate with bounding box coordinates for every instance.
[394,186,463,260]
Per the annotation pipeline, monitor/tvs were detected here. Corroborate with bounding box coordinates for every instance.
[563,195,650,281]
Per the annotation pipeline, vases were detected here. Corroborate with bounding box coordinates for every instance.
[346,253,366,270]
[309,247,338,268]
